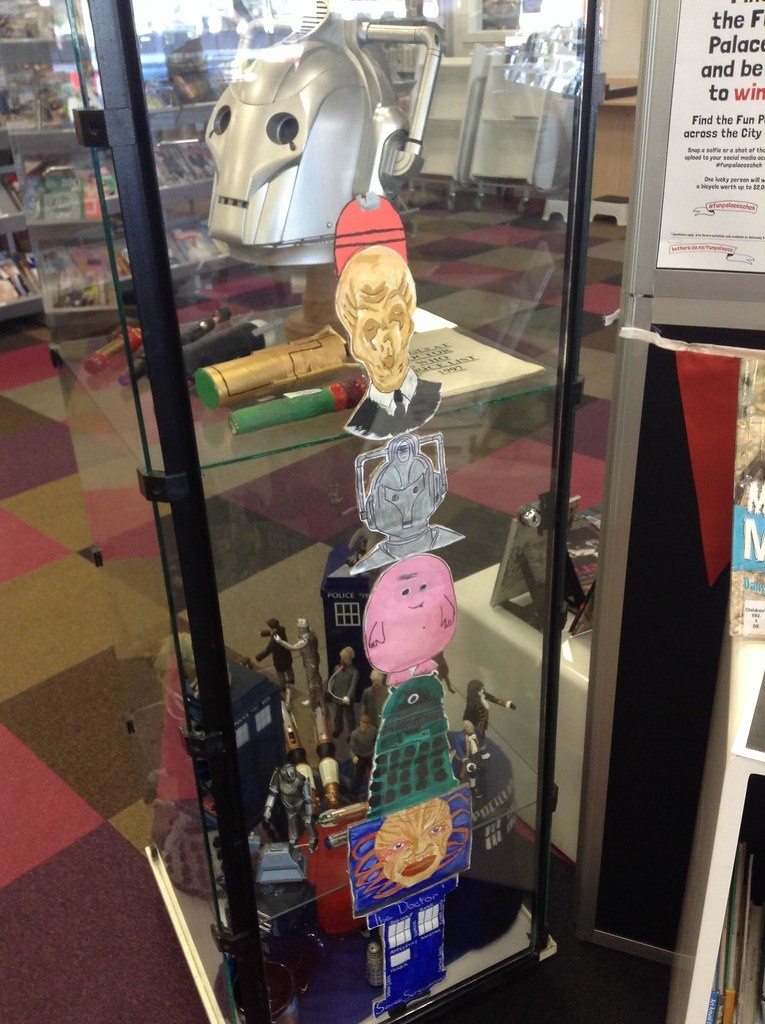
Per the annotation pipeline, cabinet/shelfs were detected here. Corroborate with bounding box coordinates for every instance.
[11,0,603,1024]
[0,29,249,320]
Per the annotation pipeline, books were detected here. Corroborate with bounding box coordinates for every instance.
[489,494,605,636]
[0,71,233,307]
[407,327,548,417]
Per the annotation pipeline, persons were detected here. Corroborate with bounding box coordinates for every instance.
[263,763,318,852]
[452,720,491,798]
[0,275,19,299]
[252,618,294,692]
[348,714,377,772]
[360,669,389,726]
[463,678,517,760]
[0,262,32,299]
[327,647,359,743]
[273,617,324,705]
